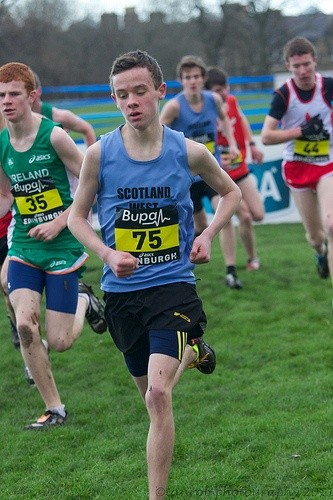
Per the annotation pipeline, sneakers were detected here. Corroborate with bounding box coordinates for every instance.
[7,315,21,348]
[188,337,217,376]
[314,238,329,278]
[23,409,69,432]
[246,257,260,271]
[78,282,108,337]
[226,273,243,291]
[26,339,48,390]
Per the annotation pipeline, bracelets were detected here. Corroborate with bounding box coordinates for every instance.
[248,142,255,146]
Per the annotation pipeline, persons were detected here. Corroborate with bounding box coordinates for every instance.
[0,62,106,429]
[65,49,244,499]
[203,67,265,272]
[31,74,95,147]
[261,37,332,280]
[0,210,49,387]
[161,56,242,289]
